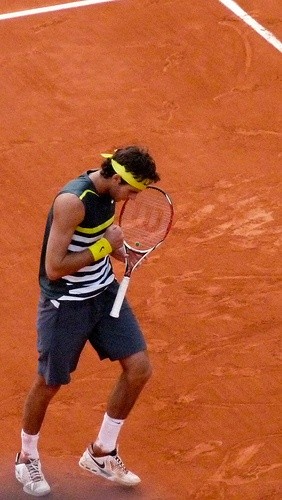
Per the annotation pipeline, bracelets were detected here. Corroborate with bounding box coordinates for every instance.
[88,237,112,261]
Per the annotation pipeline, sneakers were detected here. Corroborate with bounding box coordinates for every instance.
[14,452,51,497]
[79,444,141,487]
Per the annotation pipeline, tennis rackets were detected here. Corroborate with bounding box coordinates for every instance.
[109,185,174,318]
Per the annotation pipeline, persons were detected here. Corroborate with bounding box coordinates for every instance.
[15,144,161,496]
